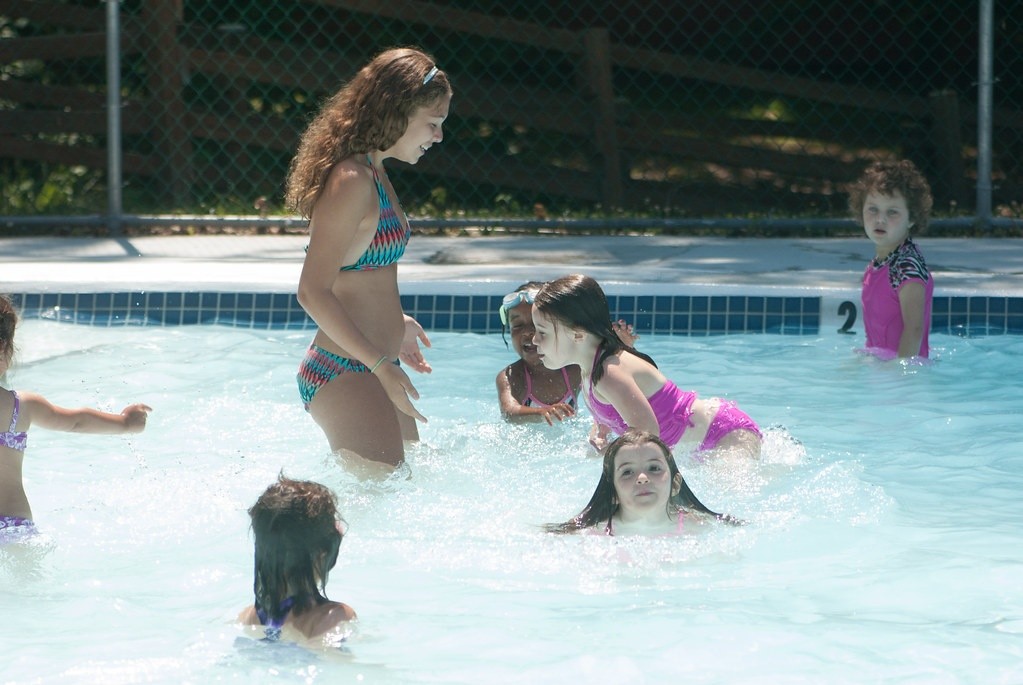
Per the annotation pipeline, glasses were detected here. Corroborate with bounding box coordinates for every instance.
[502,288,541,310]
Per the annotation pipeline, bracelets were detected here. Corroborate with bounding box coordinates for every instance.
[371,355,388,373]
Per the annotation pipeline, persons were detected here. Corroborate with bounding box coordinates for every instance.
[0,295,154,531]
[531,273,763,451]
[847,155,934,359]
[495,281,640,425]
[549,432,721,537]
[283,47,453,465]
[238,468,356,639]
